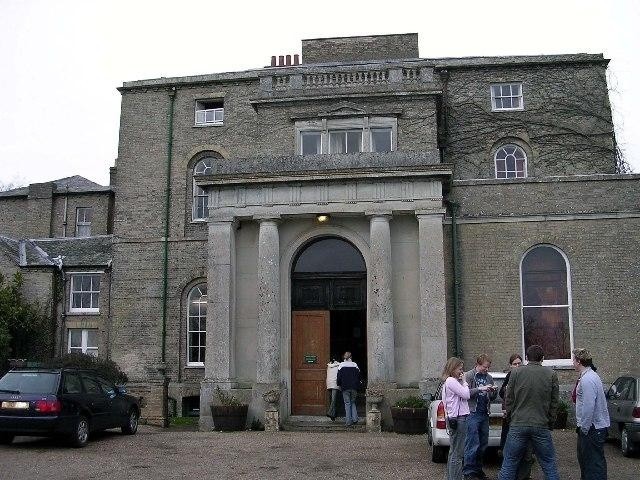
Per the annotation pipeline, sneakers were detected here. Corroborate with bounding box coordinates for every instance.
[326,414,359,427]
[464,471,489,480]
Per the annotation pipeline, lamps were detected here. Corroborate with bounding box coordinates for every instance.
[316,213,330,223]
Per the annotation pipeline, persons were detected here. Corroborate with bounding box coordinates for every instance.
[439,344,562,479]
[336,351,361,428]
[327,353,342,420]
[572,348,612,479]
[572,363,598,480]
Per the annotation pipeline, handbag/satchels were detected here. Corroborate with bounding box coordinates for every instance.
[449,419,458,429]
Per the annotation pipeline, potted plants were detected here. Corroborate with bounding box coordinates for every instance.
[554,402,568,429]
[209,385,249,431]
[389,395,428,435]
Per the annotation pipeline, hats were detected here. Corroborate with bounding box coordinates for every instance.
[572,348,592,360]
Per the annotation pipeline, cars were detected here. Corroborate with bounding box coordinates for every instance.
[601,375,640,458]
[421,371,536,465]
[0,361,142,451]
